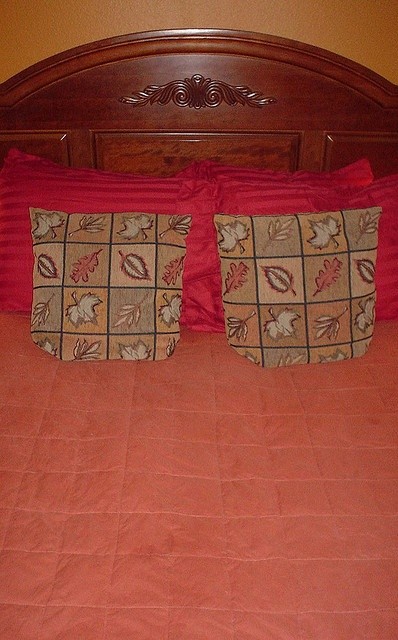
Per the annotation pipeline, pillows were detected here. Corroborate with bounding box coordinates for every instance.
[210,207,384,368]
[186,157,397,333]
[28,207,188,363]
[201,171,396,322]
[1,148,216,333]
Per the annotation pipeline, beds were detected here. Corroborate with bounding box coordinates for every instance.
[0,25,397,450]
